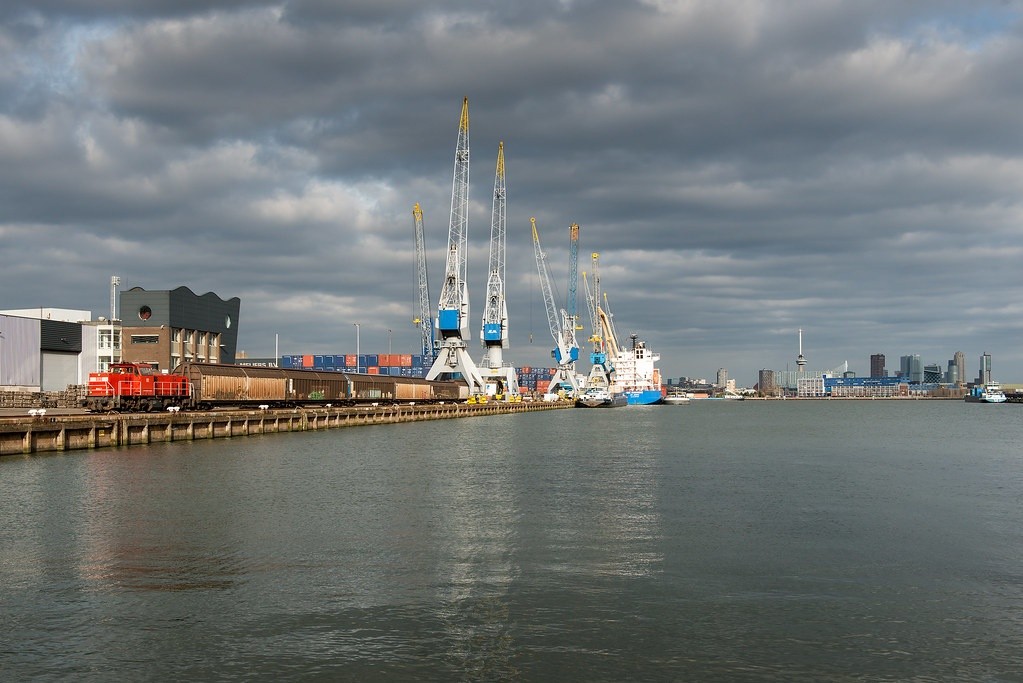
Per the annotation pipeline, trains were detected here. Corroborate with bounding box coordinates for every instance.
[83,363,504,413]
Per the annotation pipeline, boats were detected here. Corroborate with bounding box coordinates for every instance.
[663,387,691,405]
[978,381,1007,403]
[576,387,613,409]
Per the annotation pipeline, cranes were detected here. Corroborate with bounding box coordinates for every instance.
[425,96,484,397]
[412,202,435,367]
[531,217,629,397]
[476,140,519,395]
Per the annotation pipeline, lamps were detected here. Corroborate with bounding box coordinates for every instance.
[160,325,165,330]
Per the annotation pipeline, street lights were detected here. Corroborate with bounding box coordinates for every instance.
[385,329,392,376]
[354,323,359,374]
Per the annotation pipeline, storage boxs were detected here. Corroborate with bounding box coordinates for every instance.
[0,384,86,409]
[282,354,451,381]
[504,367,557,395]
